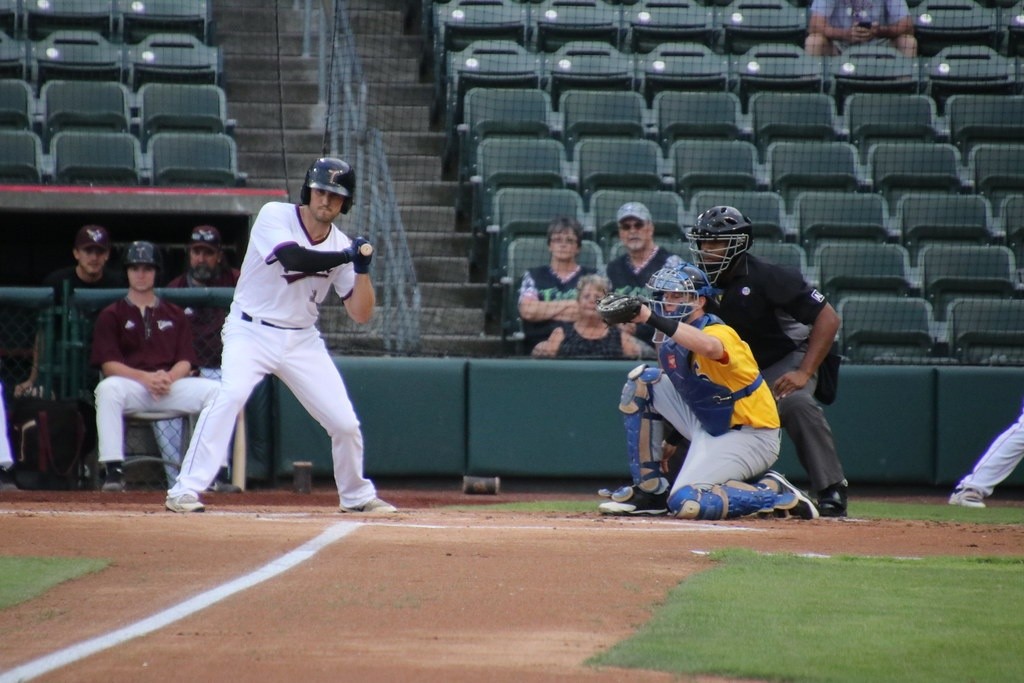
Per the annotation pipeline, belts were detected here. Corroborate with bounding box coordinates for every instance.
[242,313,303,330]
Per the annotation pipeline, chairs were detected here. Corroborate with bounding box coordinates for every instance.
[415,0,1024,368]
[0,0,250,191]
[97,370,194,471]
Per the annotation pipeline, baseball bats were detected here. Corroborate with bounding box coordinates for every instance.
[360,244,372,255]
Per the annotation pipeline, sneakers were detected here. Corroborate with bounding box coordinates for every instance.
[165,494,206,513]
[597,485,671,516]
[948,485,987,509]
[817,491,847,517]
[206,479,242,493]
[341,496,398,513]
[764,469,819,519]
[102,468,128,492]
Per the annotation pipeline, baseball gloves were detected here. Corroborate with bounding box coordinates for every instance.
[595,293,641,325]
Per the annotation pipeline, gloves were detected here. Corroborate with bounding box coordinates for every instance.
[343,237,374,274]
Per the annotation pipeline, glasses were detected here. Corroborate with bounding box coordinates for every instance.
[191,232,217,243]
[619,222,645,230]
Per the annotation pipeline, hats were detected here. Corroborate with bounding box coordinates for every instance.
[192,225,222,253]
[616,202,651,223]
[74,225,111,251]
[648,263,723,297]
[124,241,162,268]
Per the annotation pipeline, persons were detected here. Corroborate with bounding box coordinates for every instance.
[163,156,400,515]
[148,225,251,492]
[947,402,1024,508]
[658,205,849,518]
[594,255,820,521]
[13,223,130,490]
[804,0,918,82]
[89,240,244,494]
[516,201,704,361]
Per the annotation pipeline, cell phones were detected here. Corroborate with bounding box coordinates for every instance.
[858,22,871,29]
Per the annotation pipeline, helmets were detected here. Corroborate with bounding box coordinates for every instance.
[301,158,356,215]
[684,205,755,285]
